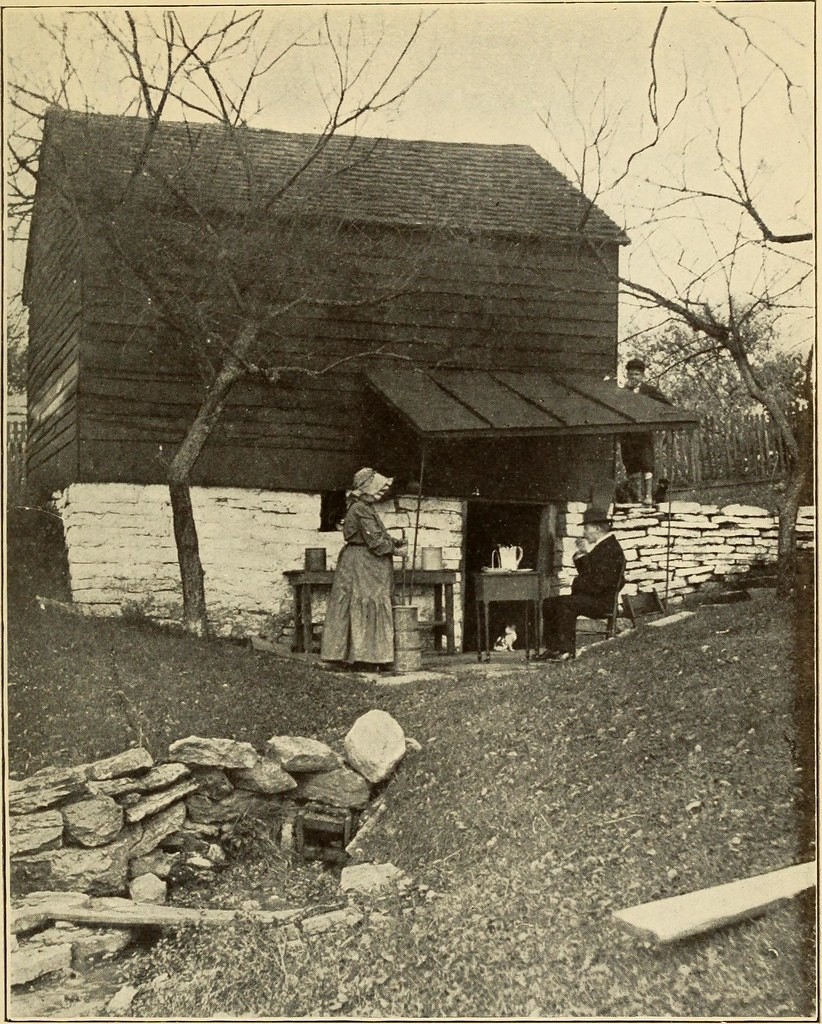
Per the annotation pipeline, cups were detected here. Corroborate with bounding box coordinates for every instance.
[499,546,524,571]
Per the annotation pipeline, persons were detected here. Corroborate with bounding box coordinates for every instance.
[318,465,409,672]
[532,507,628,664]
[618,359,665,504]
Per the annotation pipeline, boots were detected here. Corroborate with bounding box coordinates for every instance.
[630,472,653,505]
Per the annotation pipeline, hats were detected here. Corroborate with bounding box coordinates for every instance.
[345,466,393,508]
[625,358,644,371]
[576,507,615,526]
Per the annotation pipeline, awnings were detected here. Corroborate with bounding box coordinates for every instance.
[361,370,703,607]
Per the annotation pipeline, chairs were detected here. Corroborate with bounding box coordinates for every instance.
[570,558,627,659]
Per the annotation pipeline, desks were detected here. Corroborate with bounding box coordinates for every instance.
[282,568,463,654]
[473,568,541,662]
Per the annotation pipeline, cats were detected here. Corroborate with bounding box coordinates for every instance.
[493,624,518,652]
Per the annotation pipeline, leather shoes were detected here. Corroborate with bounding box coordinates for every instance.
[532,647,562,661]
[546,651,576,663]
